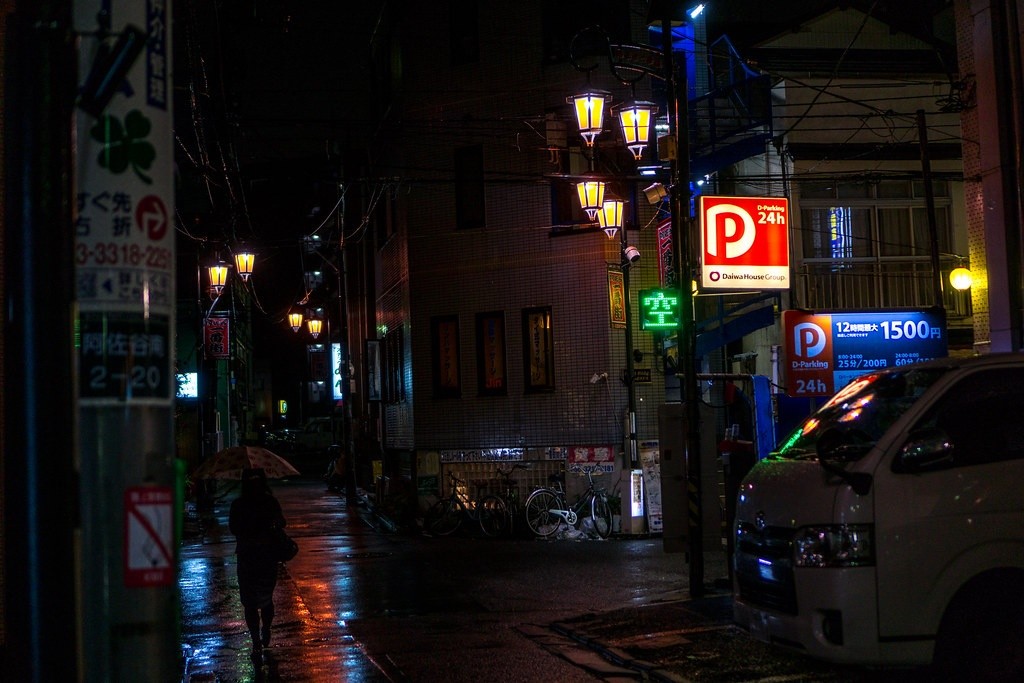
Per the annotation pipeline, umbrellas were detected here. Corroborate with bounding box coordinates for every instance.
[191,446,299,481]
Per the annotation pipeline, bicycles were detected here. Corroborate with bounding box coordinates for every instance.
[434,469,509,537]
[492,463,529,539]
[525,459,611,538]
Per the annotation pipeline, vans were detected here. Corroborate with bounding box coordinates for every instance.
[731,352,1024,682]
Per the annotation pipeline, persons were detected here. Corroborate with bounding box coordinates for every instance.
[230,465,297,656]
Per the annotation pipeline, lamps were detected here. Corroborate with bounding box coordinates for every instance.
[306,310,324,340]
[204,252,229,297]
[609,80,661,163]
[565,69,614,152]
[574,158,612,222]
[231,238,256,283]
[285,303,304,333]
[597,183,630,240]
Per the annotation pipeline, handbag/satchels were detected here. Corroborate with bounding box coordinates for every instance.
[272,538,299,561]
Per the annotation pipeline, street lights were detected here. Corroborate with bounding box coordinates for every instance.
[564,67,670,537]
[288,294,326,426]
[196,213,261,511]
